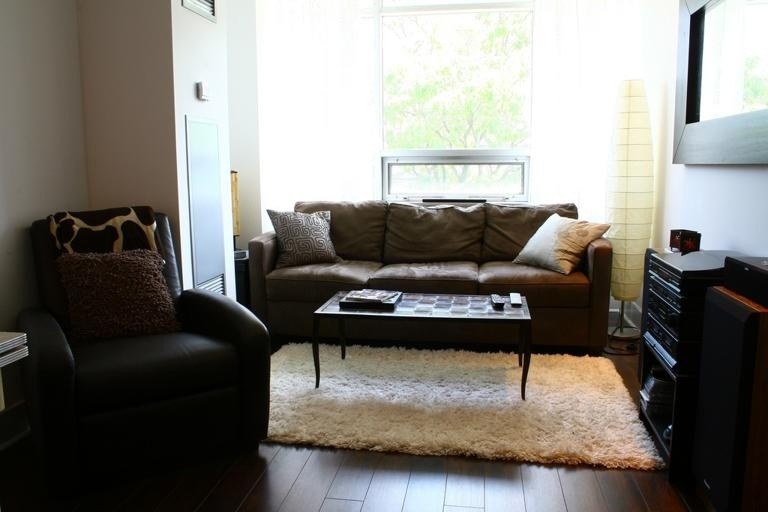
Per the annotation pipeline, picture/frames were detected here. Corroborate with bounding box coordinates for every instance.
[671,0,768,165]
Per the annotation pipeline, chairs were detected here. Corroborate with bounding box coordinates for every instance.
[20,205,269,500]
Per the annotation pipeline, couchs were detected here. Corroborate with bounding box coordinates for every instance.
[247,231,612,351]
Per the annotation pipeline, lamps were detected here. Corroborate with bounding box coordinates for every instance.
[612,81,655,350]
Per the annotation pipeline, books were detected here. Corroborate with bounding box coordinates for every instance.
[641,369,671,422]
[340,289,401,310]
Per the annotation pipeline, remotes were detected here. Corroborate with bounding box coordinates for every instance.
[509,292,524,306]
[490,292,505,313]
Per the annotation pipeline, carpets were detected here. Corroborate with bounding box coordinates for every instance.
[269,341,664,469]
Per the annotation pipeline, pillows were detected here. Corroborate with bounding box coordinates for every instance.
[483,200,580,259]
[511,210,610,275]
[295,200,387,261]
[383,203,485,262]
[57,250,174,335]
[267,209,339,267]
